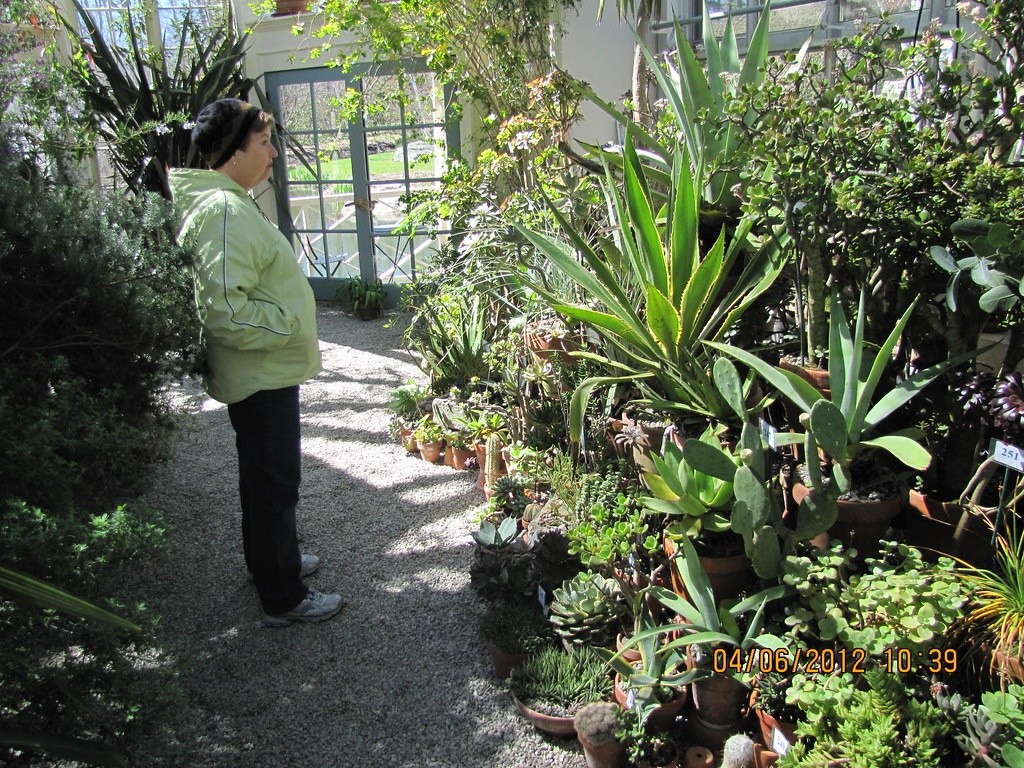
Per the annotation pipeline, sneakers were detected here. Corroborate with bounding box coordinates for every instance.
[264,587,343,627]
[298,553,321,577]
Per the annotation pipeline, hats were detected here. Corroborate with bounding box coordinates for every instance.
[190,99,259,170]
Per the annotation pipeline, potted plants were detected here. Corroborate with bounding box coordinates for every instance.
[238,0,1024,768]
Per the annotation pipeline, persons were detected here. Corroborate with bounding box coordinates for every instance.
[167,98,343,628]
